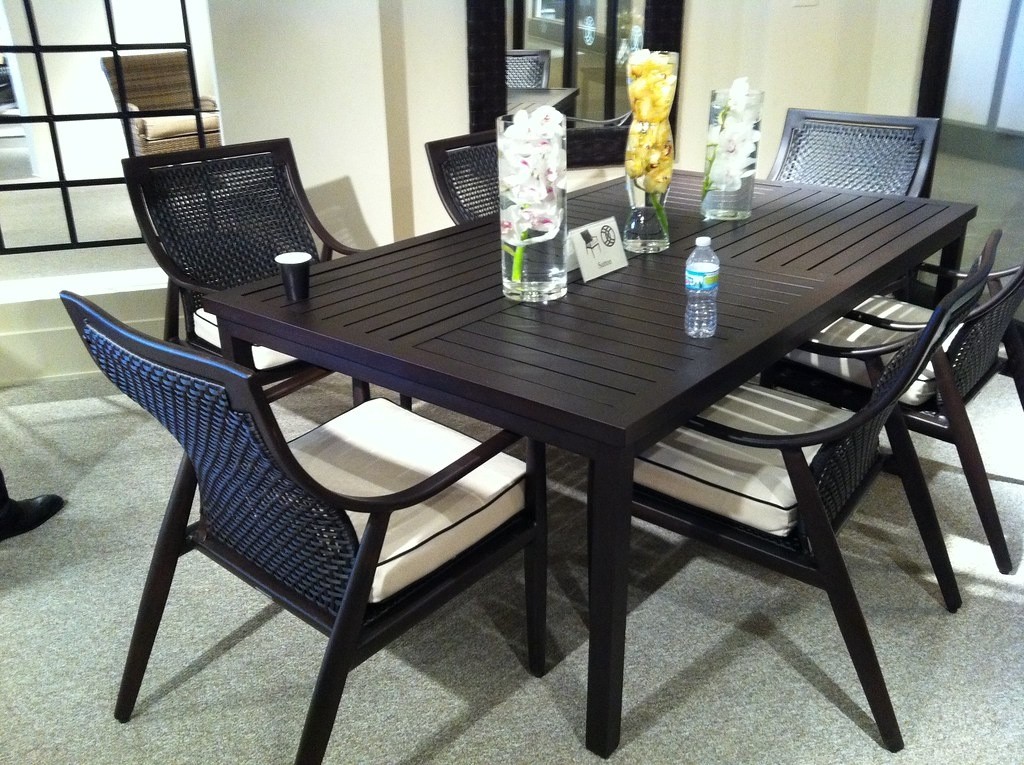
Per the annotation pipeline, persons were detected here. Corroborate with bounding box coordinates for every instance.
[0,470,65,541]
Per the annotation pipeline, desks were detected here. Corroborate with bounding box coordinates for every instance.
[508,88,580,115]
[203,168,979,761]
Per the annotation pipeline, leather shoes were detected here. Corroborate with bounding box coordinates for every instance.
[0,495,63,541]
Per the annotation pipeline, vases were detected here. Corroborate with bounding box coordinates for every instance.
[496,116,567,303]
[622,52,679,254]
[700,89,765,221]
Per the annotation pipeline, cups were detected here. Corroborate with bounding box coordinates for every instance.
[275,252,312,301]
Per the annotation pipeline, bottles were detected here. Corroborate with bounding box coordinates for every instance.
[685,237,719,338]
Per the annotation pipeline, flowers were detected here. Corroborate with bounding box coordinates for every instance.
[625,49,676,235]
[497,105,566,283]
[702,76,761,202]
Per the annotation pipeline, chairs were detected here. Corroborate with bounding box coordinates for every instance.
[506,50,550,88]
[100,51,221,157]
[121,137,411,411]
[632,229,1002,752]
[760,261,1024,574]
[61,290,547,765]
[765,107,940,198]
[425,128,499,225]
